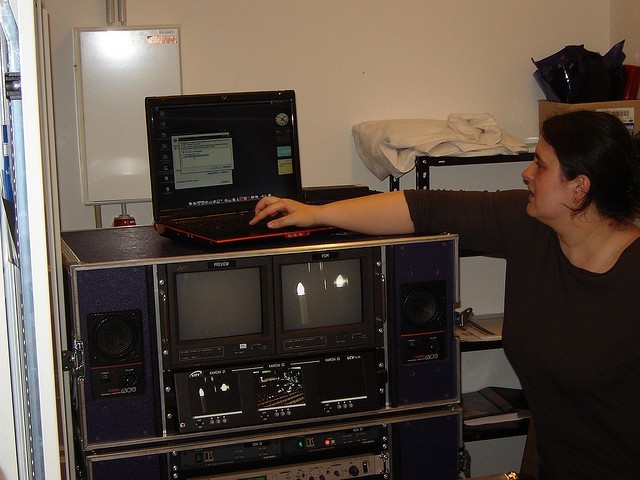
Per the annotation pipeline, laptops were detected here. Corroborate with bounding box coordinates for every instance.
[144,89,339,245]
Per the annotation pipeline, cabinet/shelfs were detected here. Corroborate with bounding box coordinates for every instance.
[394,146,609,479]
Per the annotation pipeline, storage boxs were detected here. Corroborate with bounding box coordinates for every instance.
[538,99,639,154]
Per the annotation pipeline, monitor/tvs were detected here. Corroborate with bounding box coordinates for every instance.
[312,358,368,408]
[251,362,310,419]
[174,370,247,424]
[166,255,276,366]
[277,251,373,357]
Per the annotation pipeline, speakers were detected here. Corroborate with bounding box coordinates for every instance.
[76,262,165,451]
[385,237,462,410]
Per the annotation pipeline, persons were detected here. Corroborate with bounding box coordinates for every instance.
[248,109,640,480]
